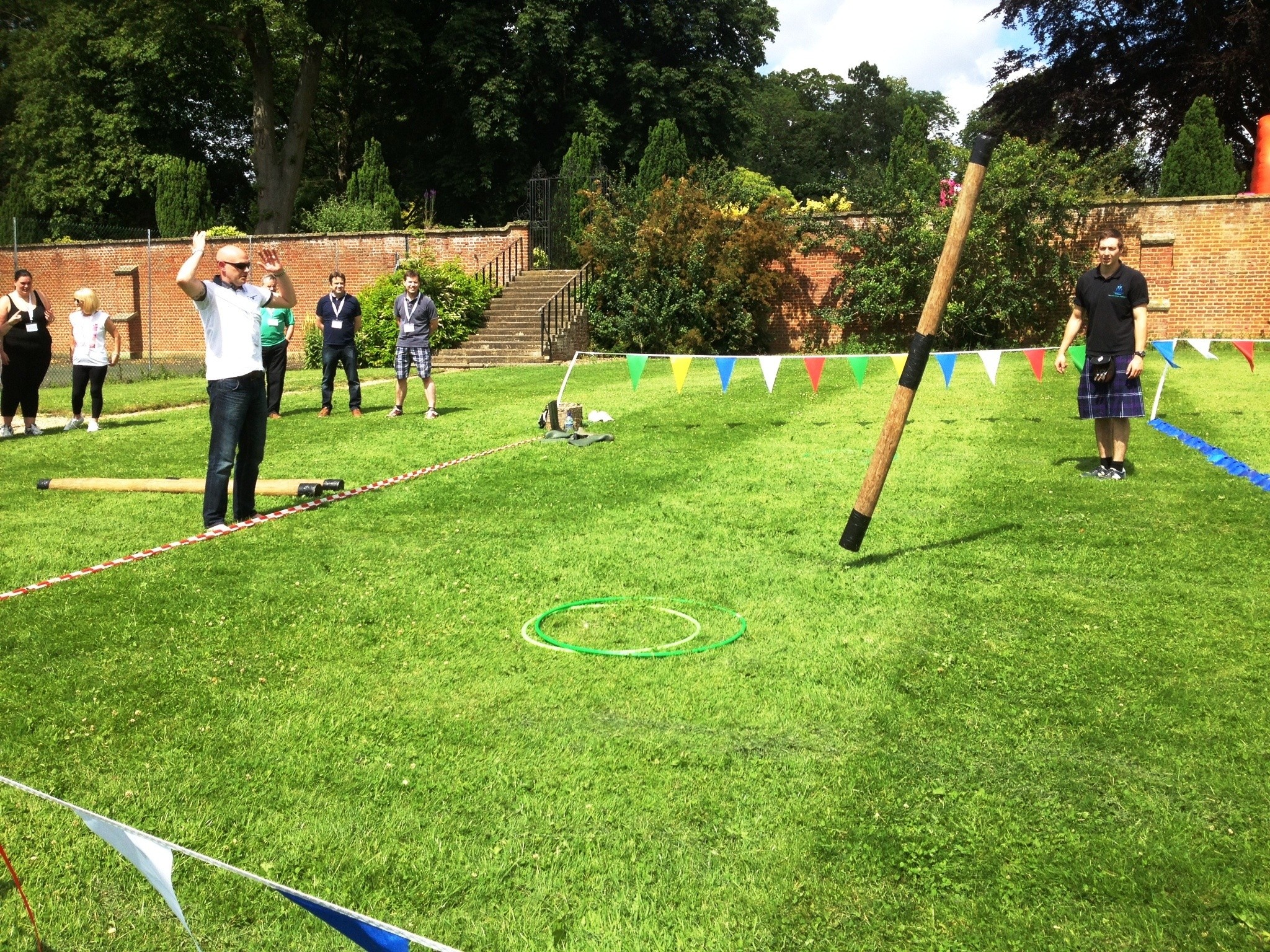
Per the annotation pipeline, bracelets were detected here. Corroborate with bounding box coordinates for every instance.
[274,269,286,277]
[7,320,13,326]
[112,353,120,358]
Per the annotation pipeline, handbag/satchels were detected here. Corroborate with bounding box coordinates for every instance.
[1090,355,1116,383]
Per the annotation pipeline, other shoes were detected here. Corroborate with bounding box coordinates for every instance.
[208,523,226,532]
[252,513,262,520]
[270,412,281,418]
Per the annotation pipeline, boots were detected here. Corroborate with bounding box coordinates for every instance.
[568,434,615,447]
[549,400,563,431]
[545,430,572,439]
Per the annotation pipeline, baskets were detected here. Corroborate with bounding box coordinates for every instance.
[545,401,583,433]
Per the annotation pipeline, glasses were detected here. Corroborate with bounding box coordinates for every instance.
[74,298,82,303]
[218,261,251,269]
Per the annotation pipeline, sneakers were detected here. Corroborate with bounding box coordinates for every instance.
[63,416,84,430]
[425,411,438,418]
[25,424,44,435]
[1102,467,1127,480]
[353,409,362,416]
[87,420,103,432]
[319,406,330,417]
[388,406,402,417]
[0,424,13,437]
[1089,465,1108,479]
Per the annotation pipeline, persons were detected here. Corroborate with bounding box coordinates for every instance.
[1054,228,1149,481]
[316,272,361,417]
[176,230,297,533]
[63,288,120,432]
[0,309,22,391]
[0,269,54,437]
[387,270,438,419]
[259,275,295,419]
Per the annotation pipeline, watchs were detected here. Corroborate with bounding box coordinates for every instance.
[192,248,204,254]
[1133,351,1146,358]
